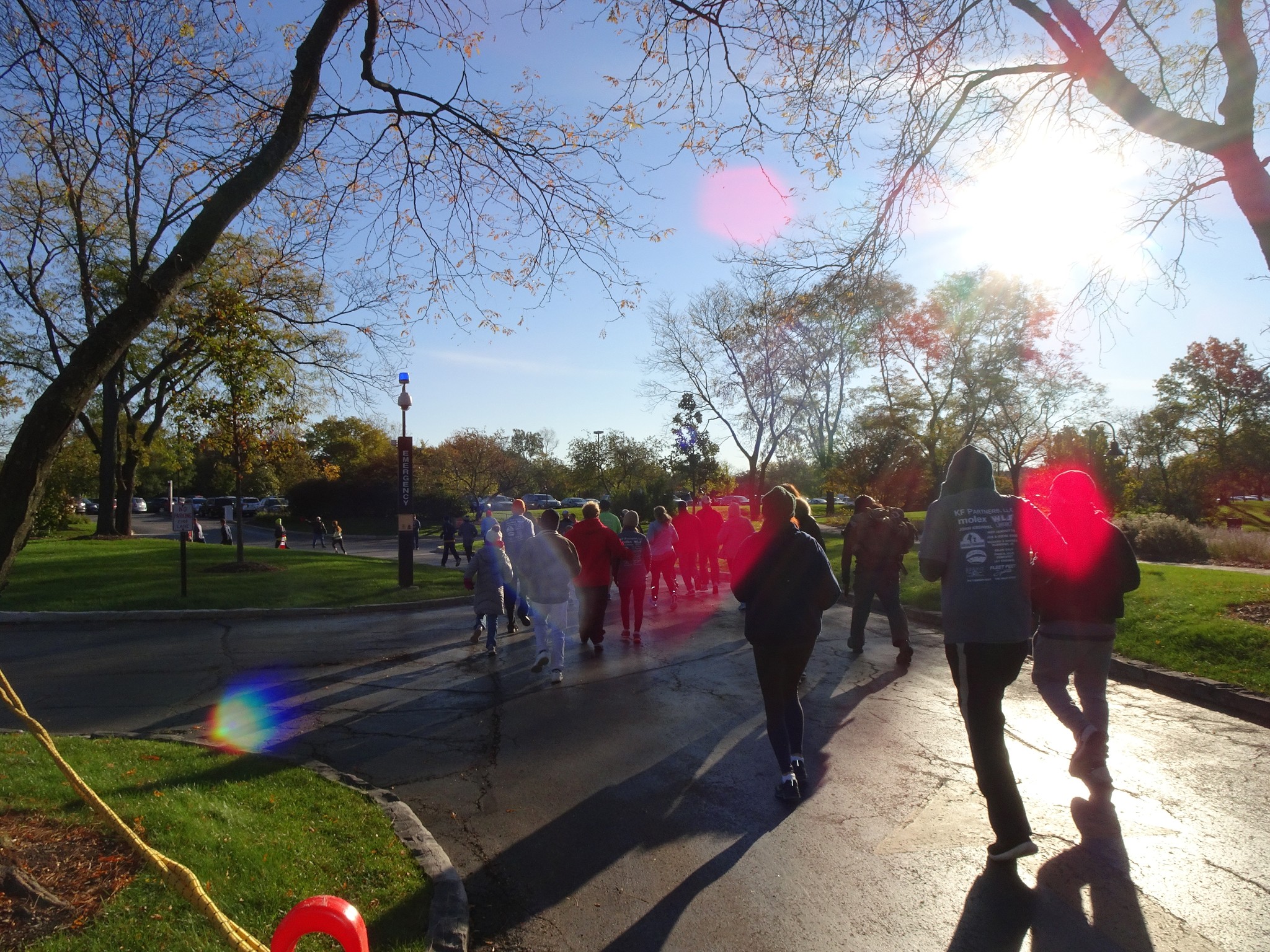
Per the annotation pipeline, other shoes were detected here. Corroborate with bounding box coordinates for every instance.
[487,645,497,655]
[1087,764,1115,784]
[456,557,461,567]
[471,626,482,643]
[551,670,563,683]
[621,631,630,638]
[792,759,808,789]
[987,836,1038,861]
[594,644,604,650]
[633,634,641,642]
[531,651,549,673]
[1069,724,1105,778]
[775,777,802,800]
[848,635,865,653]
[895,646,913,665]
[649,576,718,610]
[518,612,531,626]
[737,602,746,609]
[507,625,518,633]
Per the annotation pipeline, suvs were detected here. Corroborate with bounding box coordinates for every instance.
[521,494,561,510]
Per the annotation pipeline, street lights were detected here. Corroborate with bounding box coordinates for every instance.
[1088,420,1125,477]
[593,430,604,500]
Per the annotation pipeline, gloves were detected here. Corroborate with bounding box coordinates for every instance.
[464,578,475,590]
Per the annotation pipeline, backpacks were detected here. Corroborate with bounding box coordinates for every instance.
[857,508,916,557]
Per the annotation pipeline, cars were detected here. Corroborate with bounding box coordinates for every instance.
[801,494,855,506]
[672,492,750,506]
[471,494,515,511]
[1230,495,1270,500]
[113,495,290,517]
[585,498,601,504]
[562,497,588,508]
[70,496,99,515]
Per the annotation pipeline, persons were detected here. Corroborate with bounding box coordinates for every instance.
[612,510,652,642]
[458,515,478,563]
[193,518,206,543]
[564,501,633,649]
[220,519,233,545]
[440,516,461,566]
[840,494,914,665]
[1032,469,1140,774]
[918,445,1070,860]
[481,483,827,610]
[413,514,421,550]
[499,499,535,632]
[464,524,513,655]
[275,518,290,549]
[305,517,328,548]
[331,521,347,555]
[517,508,582,683]
[730,486,841,799]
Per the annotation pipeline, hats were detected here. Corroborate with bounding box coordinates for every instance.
[463,514,470,522]
[511,500,526,515]
[485,525,503,543]
[486,510,492,517]
[624,510,639,527]
[702,495,712,504]
[761,485,796,521]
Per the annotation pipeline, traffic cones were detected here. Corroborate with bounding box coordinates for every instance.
[278,534,287,549]
